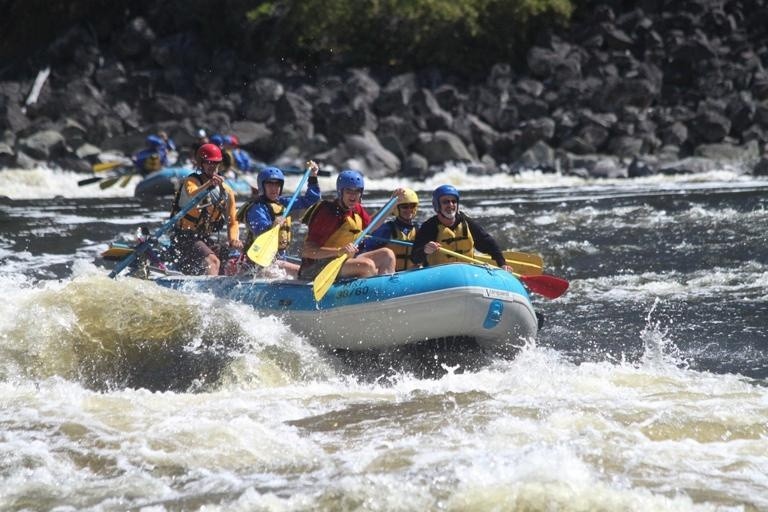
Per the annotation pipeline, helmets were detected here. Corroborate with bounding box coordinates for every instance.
[337,171,364,199]
[392,188,419,217]
[199,135,238,161]
[258,167,284,197]
[433,185,459,212]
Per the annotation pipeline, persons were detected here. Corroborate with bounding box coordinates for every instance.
[365,187,426,269]
[412,183,513,274]
[121,128,253,180]
[238,159,321,283]
[297,170,407,283]
[172,143,245,277]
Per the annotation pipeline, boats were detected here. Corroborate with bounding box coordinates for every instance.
[115,261,538,369]
[134,167,252,200]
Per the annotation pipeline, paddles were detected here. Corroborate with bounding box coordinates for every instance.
[313,196,400,303]
[222,169,258,199]
[365,235,543,275]
[78,153,154,190]
[100,246,136,260]
[246,167,312,267]
[438,235,569,298]
